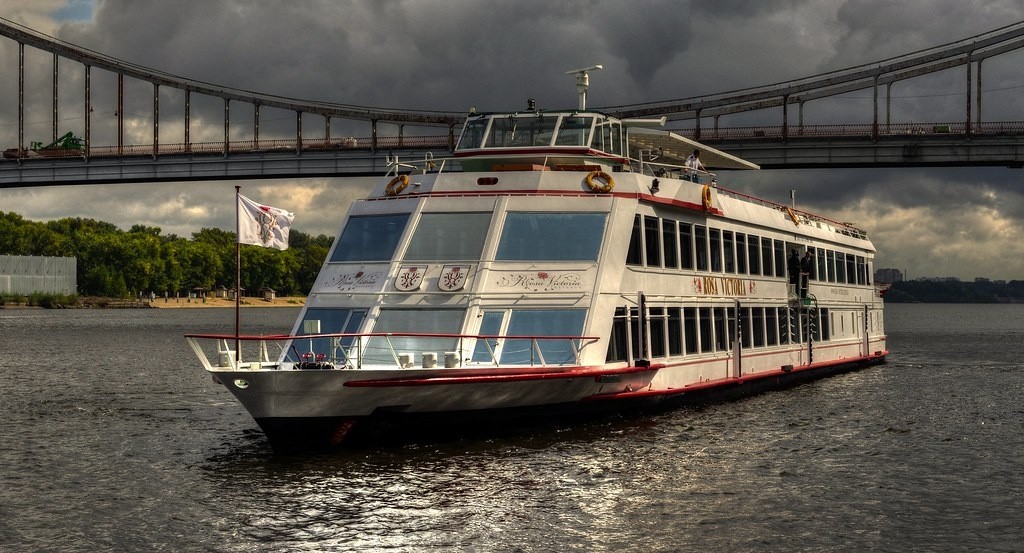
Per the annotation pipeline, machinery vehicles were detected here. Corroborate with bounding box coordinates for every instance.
[30,131,85,152]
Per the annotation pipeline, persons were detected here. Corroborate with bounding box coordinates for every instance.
[25,146,28,151]
[683,149,711,183]
[789,249,801,294]
[801,252,813,298]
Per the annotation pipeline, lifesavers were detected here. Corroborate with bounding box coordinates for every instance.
[787,207,800,226]
[385,173,410,196]
[701,184,712,209]
[585,171,616,192]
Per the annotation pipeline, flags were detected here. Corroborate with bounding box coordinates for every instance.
[237,193,295,251]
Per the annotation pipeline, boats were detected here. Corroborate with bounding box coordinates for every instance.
[182,64,888,461]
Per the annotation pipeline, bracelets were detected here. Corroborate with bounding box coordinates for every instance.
[703,169,705,171]
